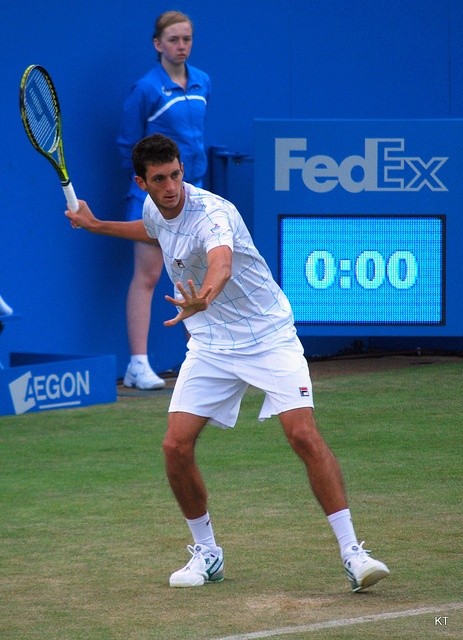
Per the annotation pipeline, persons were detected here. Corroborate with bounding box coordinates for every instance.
[64,136,391,591]
[117,10,212,391]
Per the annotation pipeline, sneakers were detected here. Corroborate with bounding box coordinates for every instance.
[169,544,226,589]
[122,363,166,391]
[340,541,391,594]
